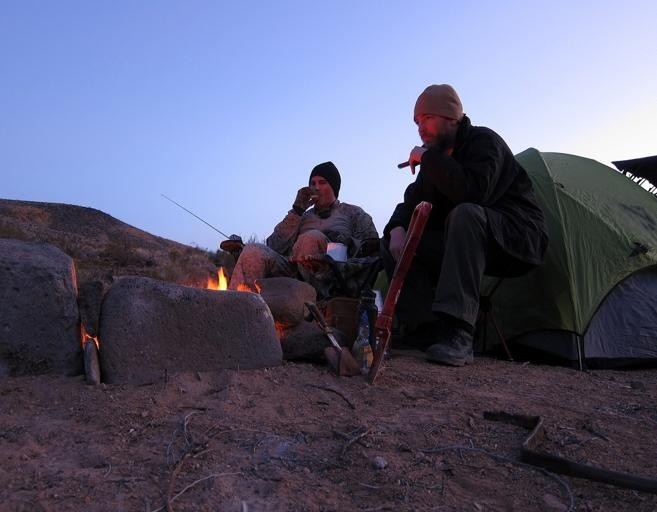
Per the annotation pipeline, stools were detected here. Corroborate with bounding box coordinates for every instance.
[472,239,540,361]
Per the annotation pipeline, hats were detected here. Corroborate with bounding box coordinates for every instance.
[413,84,463,121]
[309,161,340,196]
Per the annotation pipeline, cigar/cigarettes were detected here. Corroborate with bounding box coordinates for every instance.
[397,160,411,168]
[311,194,319,198]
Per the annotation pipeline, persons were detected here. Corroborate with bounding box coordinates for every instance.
[229,161,380,301]
[381,84,548,367]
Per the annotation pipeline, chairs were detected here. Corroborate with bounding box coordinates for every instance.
[326,237,384,348]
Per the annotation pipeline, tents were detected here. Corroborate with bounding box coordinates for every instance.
[369,148,657,373]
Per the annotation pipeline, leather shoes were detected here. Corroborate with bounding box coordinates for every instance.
[424,332,473,367]
